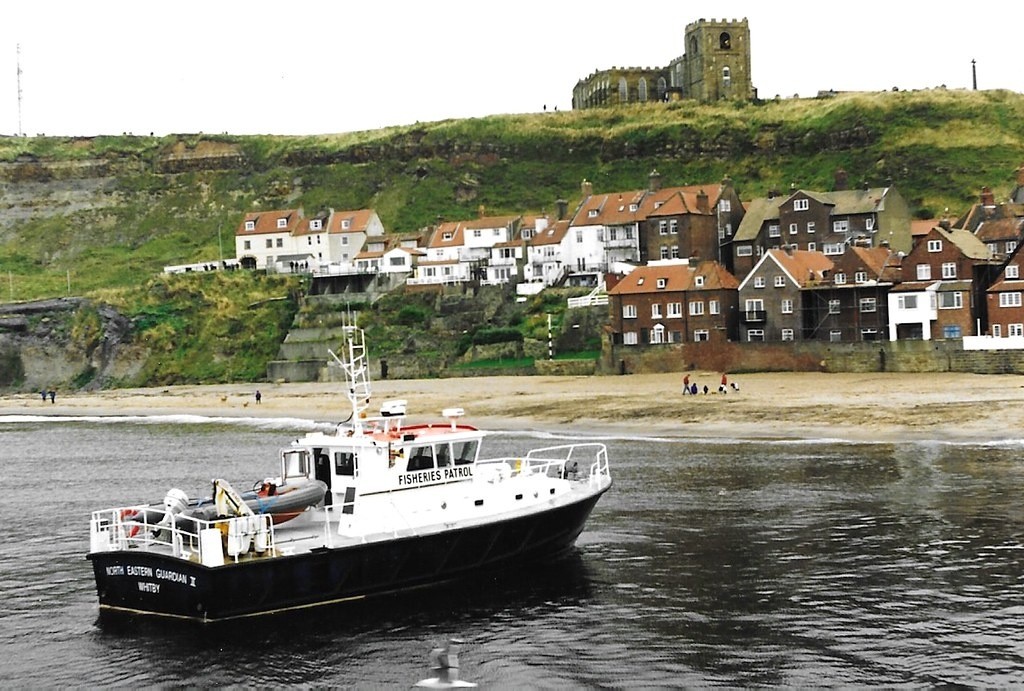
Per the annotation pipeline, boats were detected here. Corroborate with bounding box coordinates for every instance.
[85,299,613,642]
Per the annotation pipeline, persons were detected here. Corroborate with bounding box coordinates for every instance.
[255,390,262,404]
[42,390,47,401]
[50,389,56,404]
[682,371,741,397]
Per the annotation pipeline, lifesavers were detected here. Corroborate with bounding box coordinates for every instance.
[116,510,141,537]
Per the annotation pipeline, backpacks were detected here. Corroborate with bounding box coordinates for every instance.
[719,387,723,391]
